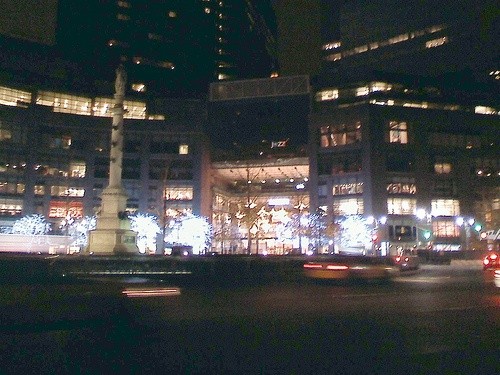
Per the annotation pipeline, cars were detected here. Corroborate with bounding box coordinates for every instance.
[303,243,500,291]
